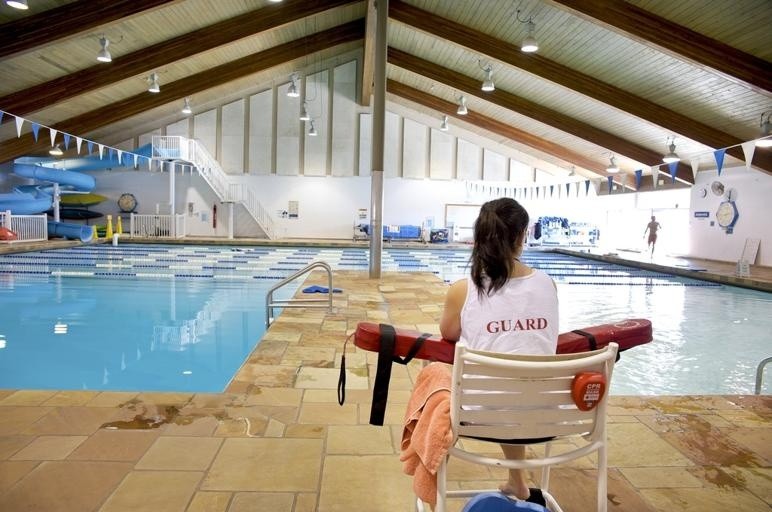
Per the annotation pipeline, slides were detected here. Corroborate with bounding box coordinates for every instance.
[0,143,152,243]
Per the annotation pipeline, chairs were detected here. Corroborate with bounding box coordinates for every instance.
[416,341,620,511]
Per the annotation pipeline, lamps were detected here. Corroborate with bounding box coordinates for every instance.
[288,79,319,139]
[438,64,494,132]
[662,132,682,164]
[605,152,622,175]
[48,143,64,157]
[95,35,119,62]
[148,69,193,117]
[516,13,540,52]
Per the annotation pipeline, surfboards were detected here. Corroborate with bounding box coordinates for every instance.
[50,193,108,205]
[41,209,103,220]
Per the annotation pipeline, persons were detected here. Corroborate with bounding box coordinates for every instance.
[644,215,662,254]
[439,198,559,500]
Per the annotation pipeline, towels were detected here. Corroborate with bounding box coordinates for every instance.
[400,360,455,509]
